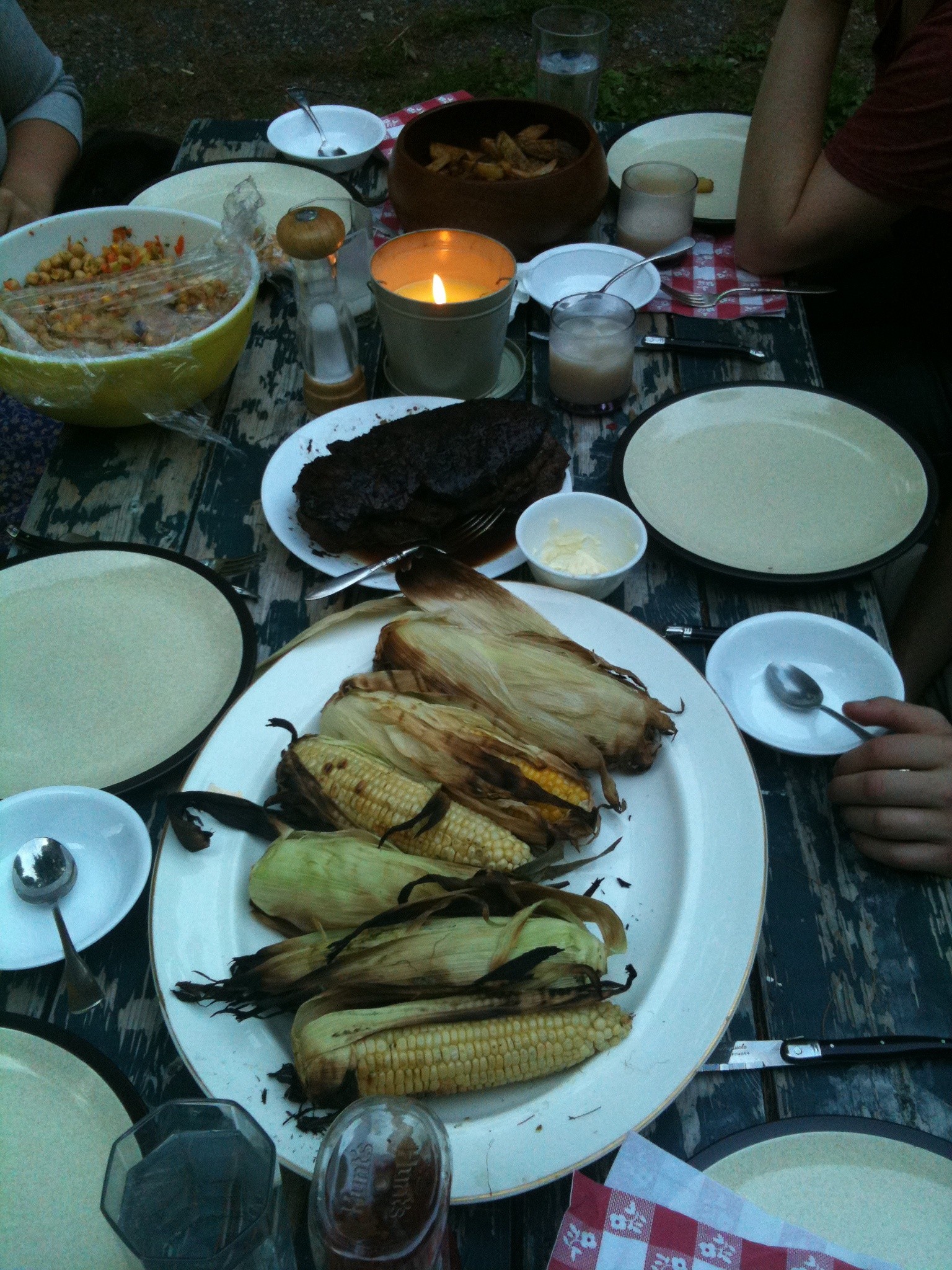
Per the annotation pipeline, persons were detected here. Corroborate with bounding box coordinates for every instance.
[1,0,176,237]
[827,516,950,875]
[734,1,948,393]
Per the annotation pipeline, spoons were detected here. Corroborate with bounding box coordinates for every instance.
[12,837,105,1017]
[555,236,695,311]
[767,661,910,775]
[283,83,348,156]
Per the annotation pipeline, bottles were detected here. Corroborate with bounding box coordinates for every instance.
[278,208,371,410]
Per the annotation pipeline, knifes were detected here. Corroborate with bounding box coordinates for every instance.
[529,330,774,364]
[696,1033,952,1073]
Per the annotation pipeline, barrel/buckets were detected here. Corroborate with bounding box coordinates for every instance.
[366,224,518,400]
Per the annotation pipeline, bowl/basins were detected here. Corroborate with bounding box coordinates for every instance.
[265,105,386,173]
[523,244,662,317]
[0,785,152,971]
[705,608,908,754]
[514,491,648,599]
[389,97,610,262]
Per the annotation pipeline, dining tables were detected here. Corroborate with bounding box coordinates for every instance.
[2,110,952,1270]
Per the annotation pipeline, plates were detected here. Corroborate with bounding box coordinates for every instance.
[261,396,573,592]
[0,1009,162,1268]
[128,156,361,275]
[612,377,938,583]
[607,109,753,224]
[688,1110,951,1269]
[0,539,258,800]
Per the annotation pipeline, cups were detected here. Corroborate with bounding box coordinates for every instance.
[285,193,380,321]
[548,291,638,416]
[616,160,698,268]
[104,1096,298,1269]
[533,5,610,125]
[142,576,773,1219]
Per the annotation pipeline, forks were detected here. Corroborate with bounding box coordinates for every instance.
[659,281,838,308]
[5,523,265,576]
[301,507,508,599]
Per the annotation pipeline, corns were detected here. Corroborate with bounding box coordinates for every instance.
[154,537,686,1103]
